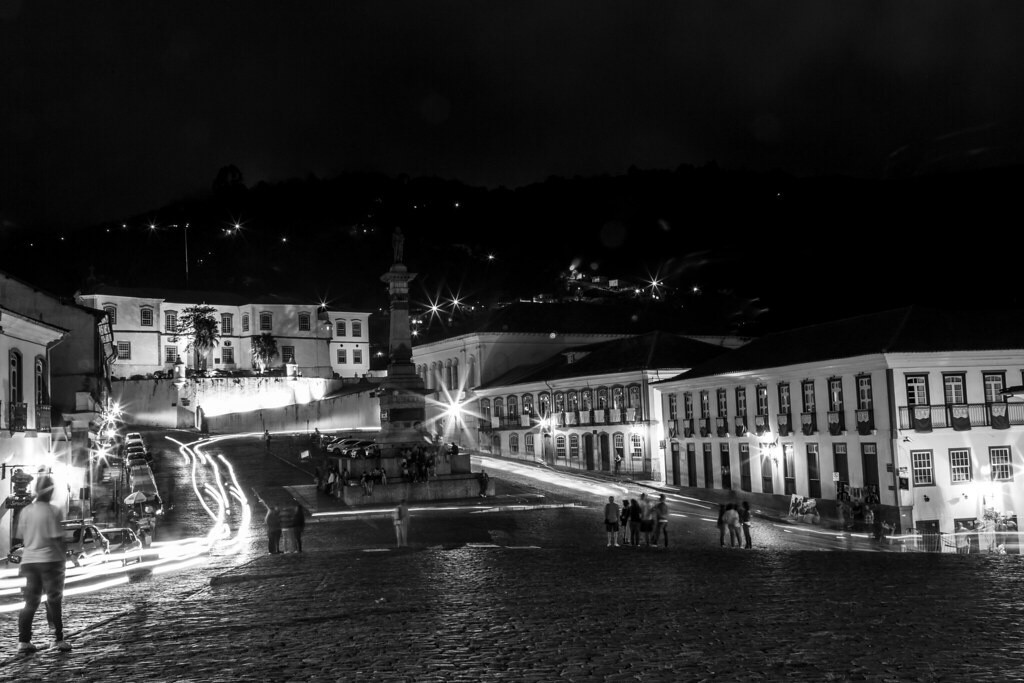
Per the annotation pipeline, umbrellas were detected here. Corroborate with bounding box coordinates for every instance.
[123,489,156,519]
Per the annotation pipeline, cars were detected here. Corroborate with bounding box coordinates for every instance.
[320,435,377,457]
[124,431,164,521]
[6,524,110,568]
[99,528,143,564]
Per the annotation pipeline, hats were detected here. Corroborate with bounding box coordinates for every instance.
[720,505,725,507]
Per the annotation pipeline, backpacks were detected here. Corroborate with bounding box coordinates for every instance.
[393,507,405,520]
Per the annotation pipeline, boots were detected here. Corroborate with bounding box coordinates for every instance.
[337,491,340,498]
[745,538,749,549]
[749,537,752,549]
[607,532,612,547]
[614,532,621,547]
[330,486,334,494]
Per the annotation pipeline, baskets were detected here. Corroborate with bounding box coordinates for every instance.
[268,436,272,439]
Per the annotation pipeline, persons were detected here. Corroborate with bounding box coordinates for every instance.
[479,469,489,498]
[15,477,72,652]
[651,494,670,548]
[604,497,622,547]
[620,500,630,544]
[308,428,462,498]
[264,430,271,447]
[74,499,157,544]
[393,499,411,547]
[265,504,286,555]
[291,505,305,554]
[614,454,622,475]
[626,499,641,547]
[717,500,753,550]
[639,494,653,548]
[789,480,1022,555]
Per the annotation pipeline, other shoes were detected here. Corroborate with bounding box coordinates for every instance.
[18,641,36,653]
[638,544,640,546]
[50,641,72,650]
[650,544,657,547]
[277,551,284,553]
[480,494,485,497]
[370,493,372,497]
[731,547,735,549]
[271,552,279,554]
[484,495,486,498]
[368,493,370,496]
[299,550,302,552]
[738,547,743,549]
[294,551,300,554]
